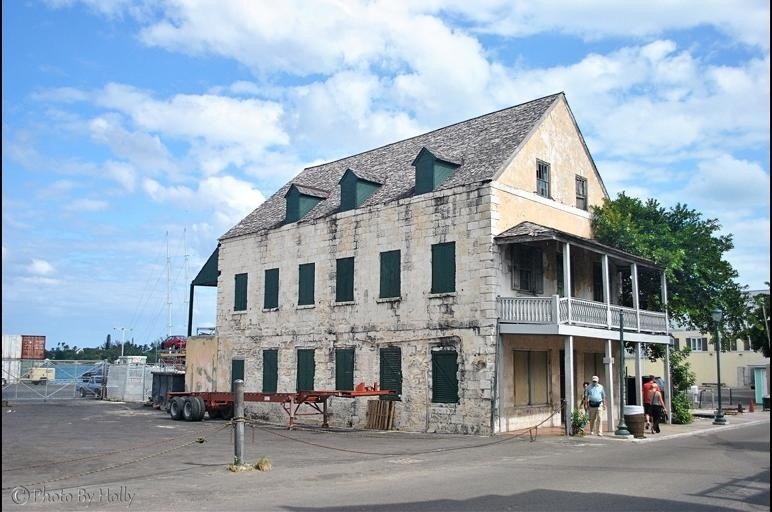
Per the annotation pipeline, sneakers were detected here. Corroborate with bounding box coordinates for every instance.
[646,423,659,433]
[590,431,603,436]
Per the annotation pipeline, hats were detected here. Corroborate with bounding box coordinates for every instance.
[592,376,599,381]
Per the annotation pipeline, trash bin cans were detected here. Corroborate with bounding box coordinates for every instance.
[762,397,770,411]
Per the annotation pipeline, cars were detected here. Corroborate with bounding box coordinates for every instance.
[161,334,187,348]
[160,357,185,368]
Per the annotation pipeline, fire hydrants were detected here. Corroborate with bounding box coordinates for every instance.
[748,399,755,412]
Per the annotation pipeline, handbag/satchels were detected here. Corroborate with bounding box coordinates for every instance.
[589,400,600,407]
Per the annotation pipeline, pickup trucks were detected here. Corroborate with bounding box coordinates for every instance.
[75,374,104,398]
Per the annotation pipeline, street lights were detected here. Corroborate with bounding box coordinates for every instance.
[113,326,134,356]
[709,307,727,426]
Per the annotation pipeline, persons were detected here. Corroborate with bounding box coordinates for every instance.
[644,375,660,430]
[579,382,589,414]
[655,377,665,404]
[647,383,669,435]
[584,375,608,437]
